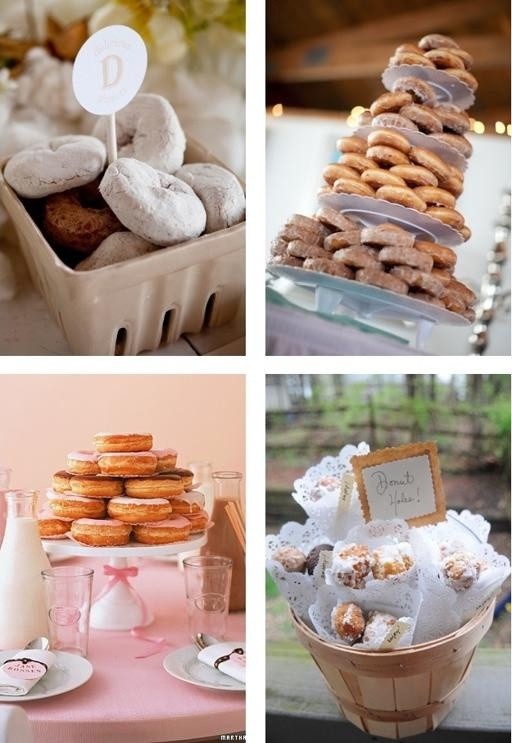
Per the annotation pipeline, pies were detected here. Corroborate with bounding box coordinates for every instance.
[36,433,209,545]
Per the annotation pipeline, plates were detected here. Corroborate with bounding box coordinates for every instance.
[162,642,244,696]
[313,188,466,255]
[2,648,94,705]
[343,119,469,179]
[268,259,472,328]
[379,63,478,114]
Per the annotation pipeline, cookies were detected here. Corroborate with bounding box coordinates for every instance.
[265,33,479,323]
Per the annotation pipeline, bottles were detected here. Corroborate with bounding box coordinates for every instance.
[2,490,49,650]
[200,467,244,613]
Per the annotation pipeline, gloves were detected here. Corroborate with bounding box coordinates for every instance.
[1,130,246,356]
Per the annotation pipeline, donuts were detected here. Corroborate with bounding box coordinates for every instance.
[3,92,246,270]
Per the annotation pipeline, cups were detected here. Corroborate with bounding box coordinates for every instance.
[181,554,235,637]
[40,562,95,658]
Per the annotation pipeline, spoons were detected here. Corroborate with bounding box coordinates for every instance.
[3,635,50,665]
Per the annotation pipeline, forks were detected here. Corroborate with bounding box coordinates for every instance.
[189,632,223,659]
